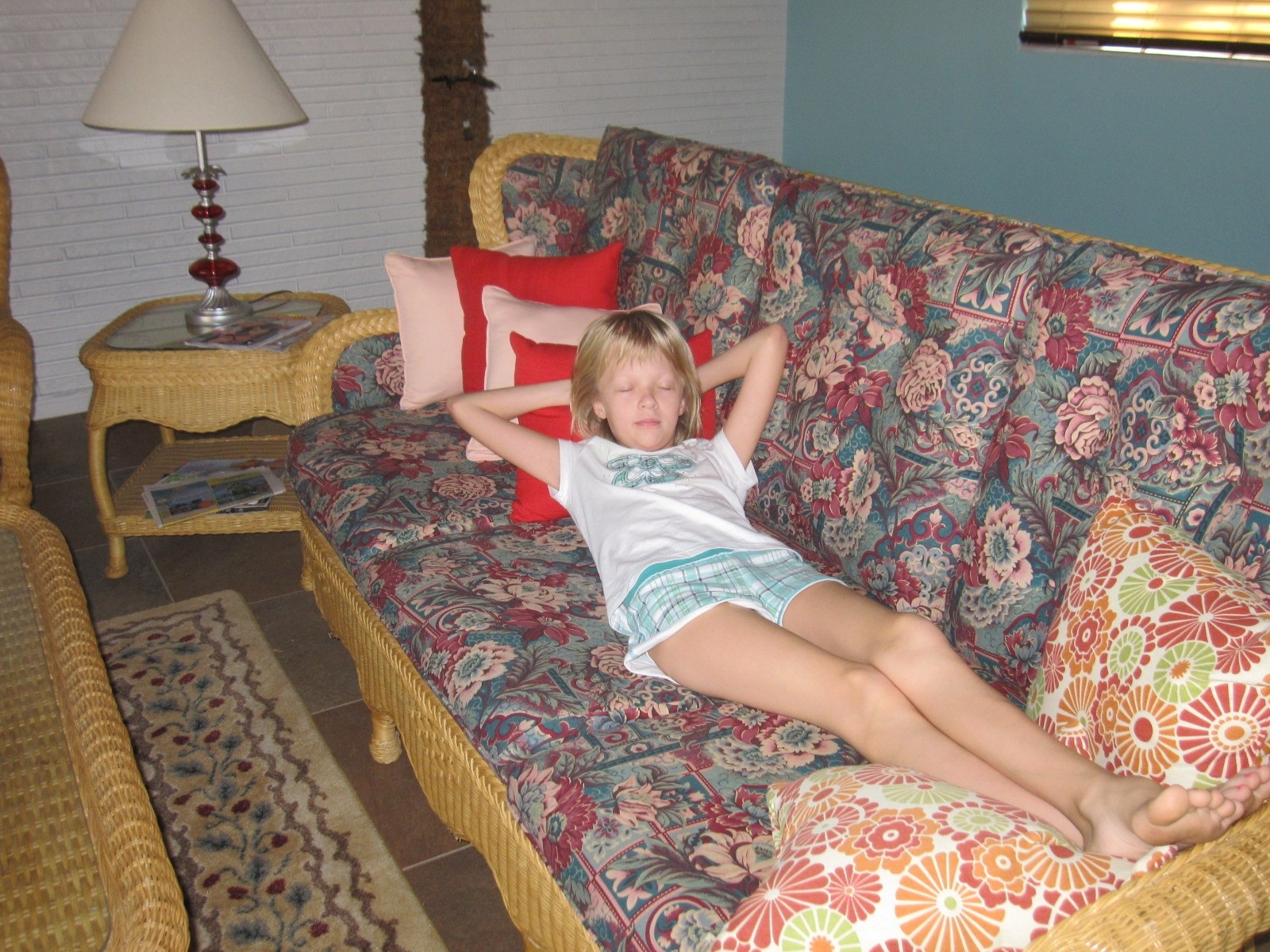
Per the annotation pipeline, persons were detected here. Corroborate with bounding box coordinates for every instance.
[448,310,1270,857]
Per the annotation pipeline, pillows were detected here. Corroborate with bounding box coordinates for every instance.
[509,330,715,522]
[712,764,1177,952]
[450,241,622,394]
[1024,490,1270,790]
[466,285,663,461]
[384,235,538,409]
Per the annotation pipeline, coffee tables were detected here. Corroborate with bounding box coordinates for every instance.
[80,292,351,592]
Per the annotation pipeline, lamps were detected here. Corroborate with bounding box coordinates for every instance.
[81,0,308,327]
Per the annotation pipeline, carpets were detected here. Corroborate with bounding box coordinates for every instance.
[92,591,446,952]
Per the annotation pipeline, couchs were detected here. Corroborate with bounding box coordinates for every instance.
[301,127,1270,952]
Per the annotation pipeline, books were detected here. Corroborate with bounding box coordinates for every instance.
[184,313,337,351]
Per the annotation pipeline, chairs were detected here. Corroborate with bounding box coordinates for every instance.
[0,158,189,952]
